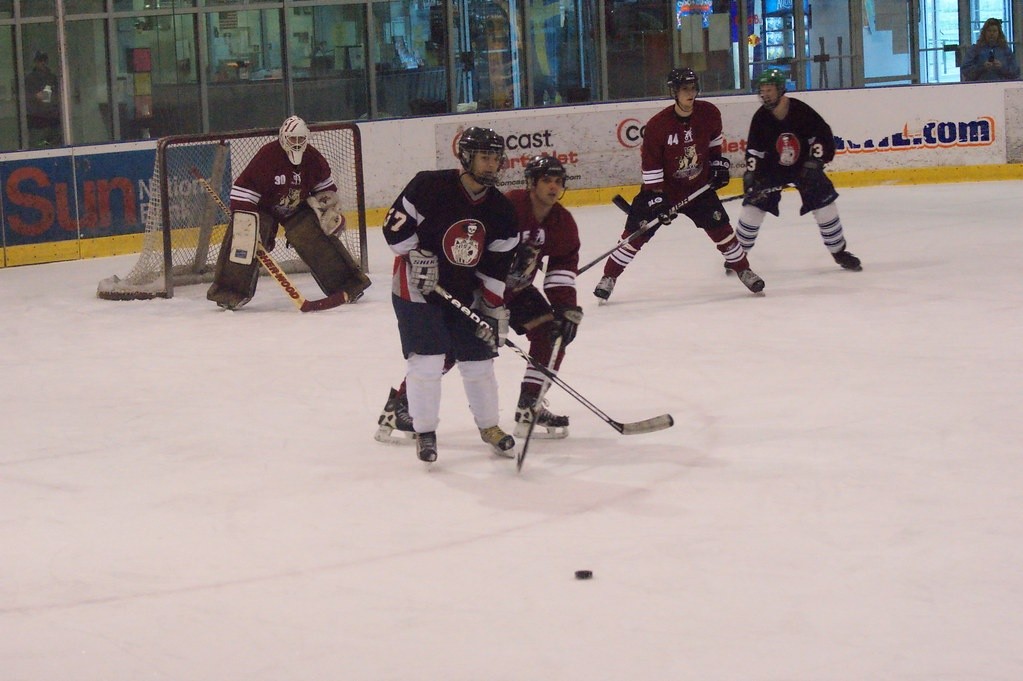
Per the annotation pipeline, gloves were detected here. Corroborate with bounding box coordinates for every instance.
[549,304,584,350]
[800,159,824,190]
[742,171,770,206]
[408,245,440,297]
[475,300,509,353]
[706,156,731,189]
[640,190,678,225]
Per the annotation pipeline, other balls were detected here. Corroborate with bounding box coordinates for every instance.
[574,570,593,580]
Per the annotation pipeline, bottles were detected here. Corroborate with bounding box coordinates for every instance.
[42,85,52,103]
[555,92,562,105]
[543,90,550,106]
[141,104,151,139]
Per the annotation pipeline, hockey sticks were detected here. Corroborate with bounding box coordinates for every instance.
[612,183,797,216]
[434,284,674,436]
[517,333,564,473]
[538,182,713,277]
[191,165,349,313]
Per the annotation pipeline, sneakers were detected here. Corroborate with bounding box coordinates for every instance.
[594,273,615,306]
[375,387,413,445]
[724,260,734,278]
[512,383,570,441]
[832,247,864,273]
[478,424,515,459]
[738,266,765,298]
[415,430,437,470]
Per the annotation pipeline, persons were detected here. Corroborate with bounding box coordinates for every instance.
[723,68,863,276]
[593,67,765,306]
[381,127,523,470]
[206,116,372,311]
[25,50,63,145]
[373,155,584,446]
[960,18,1020,82]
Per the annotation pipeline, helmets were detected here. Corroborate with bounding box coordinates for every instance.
[279,114,310,165]
[667,67,701,92]
[458,128,503,186]
[524,154,566,188]
[755,69,785,111]
